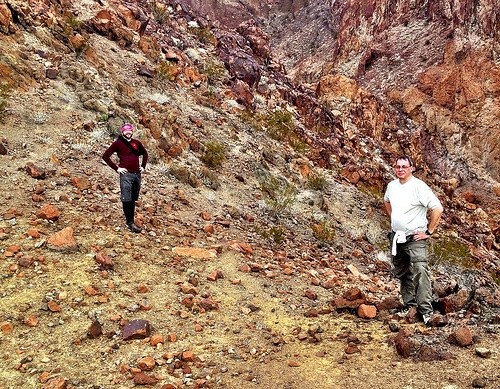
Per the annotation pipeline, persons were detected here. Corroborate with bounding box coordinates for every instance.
[102,123,148,233]
[384,154,444,325]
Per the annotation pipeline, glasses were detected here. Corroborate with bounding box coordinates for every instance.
[395,165,409,171]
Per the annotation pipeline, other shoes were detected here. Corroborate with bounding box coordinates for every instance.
[125,221,143,233]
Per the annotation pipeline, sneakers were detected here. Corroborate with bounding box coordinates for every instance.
[395,306,411,317]
[423,313,432,324]
